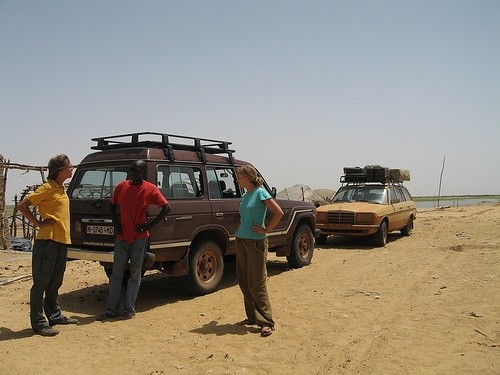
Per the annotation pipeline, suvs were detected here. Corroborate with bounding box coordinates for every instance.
[66,131,317,296]
[315,176,417,247]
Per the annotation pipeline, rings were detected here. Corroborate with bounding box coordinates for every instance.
[141,229,143,231]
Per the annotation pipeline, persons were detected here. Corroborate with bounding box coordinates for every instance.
[96,160,171,320]
[18,154,78,335]
[234,166,284,336]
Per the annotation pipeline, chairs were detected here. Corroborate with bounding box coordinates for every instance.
[171,184,196,198]
[208,180,234,200]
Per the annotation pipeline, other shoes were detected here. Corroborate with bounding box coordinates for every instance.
[95,312,115,320]
[49,316,79,325]
[244,319,255,324]
[33,325,60,336]
[118,312,131,319]
[261,326,272,335]
